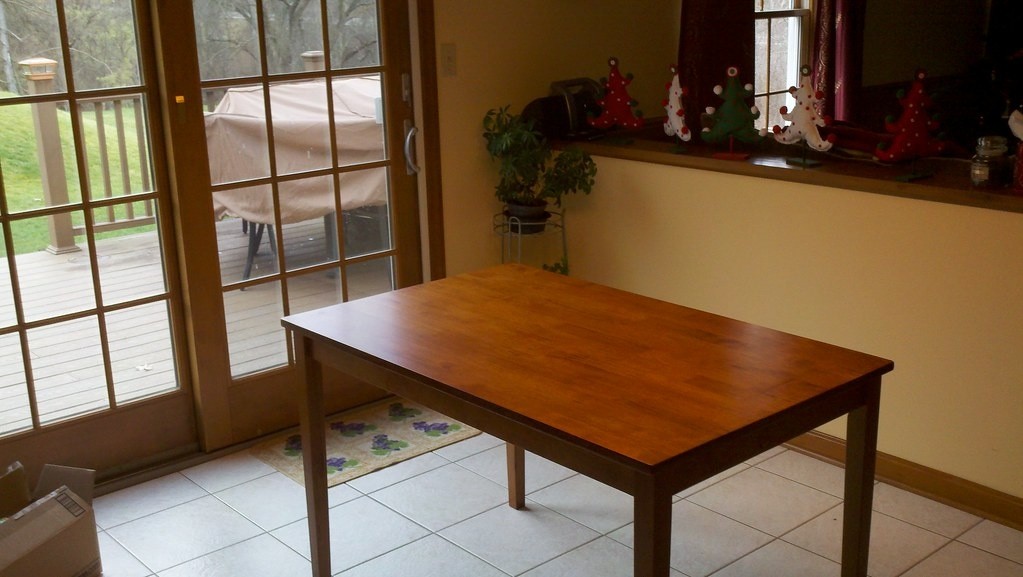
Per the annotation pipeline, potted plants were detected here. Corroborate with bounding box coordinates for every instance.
[482,103,598,233]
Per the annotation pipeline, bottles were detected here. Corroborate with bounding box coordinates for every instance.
[971,135,1011,190]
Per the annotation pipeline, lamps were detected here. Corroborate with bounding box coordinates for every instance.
[15,55,59,82]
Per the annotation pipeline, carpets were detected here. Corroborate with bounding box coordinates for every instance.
[249,398,482,489]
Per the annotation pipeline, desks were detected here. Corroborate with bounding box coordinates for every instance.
[281,263,895,577]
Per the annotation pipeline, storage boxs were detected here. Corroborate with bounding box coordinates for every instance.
[0,461,104,577]
